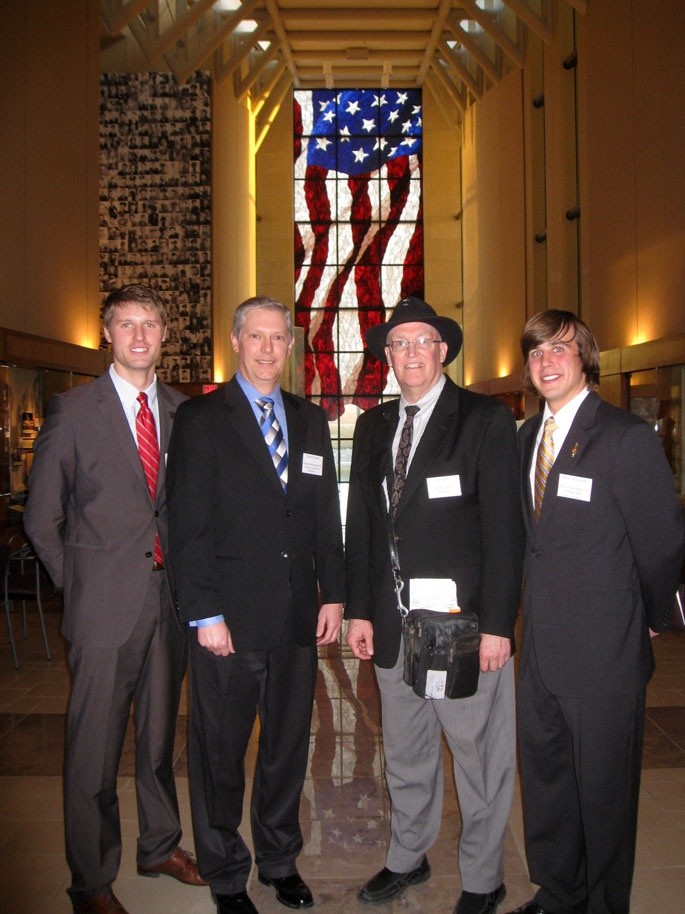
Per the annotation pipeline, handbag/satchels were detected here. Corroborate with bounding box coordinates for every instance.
[403,609,482,699]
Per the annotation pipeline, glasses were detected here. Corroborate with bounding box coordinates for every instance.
[383,339,441,350]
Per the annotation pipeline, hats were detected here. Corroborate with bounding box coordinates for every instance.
[366,298,464,367]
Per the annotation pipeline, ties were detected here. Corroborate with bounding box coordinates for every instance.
[534,417,559,526]
[255,396,289,495]
[391,406,420,523]
[136,391,164,564]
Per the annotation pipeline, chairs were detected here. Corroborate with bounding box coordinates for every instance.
[0,493,57,670]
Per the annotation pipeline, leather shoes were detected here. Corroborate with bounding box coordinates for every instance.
[258,871,313,909]
[212,890,259,914]
[358,853,431,905]
[137,848,205,886]
[453,883,507,914]
[74,888,129,914]
[506,901,549,914]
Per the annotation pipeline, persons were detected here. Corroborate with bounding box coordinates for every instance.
[507,310,685,913]
[344,297,525,914]
[166,297,343,914]
[22,283,210,914]
